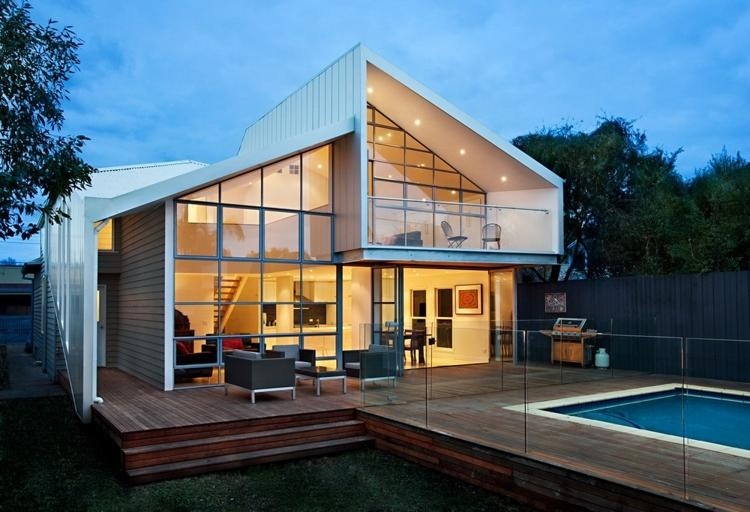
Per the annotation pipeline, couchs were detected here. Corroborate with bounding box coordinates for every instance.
[202,333,266,362]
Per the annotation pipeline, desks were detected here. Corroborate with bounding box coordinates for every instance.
[374,329,432,360]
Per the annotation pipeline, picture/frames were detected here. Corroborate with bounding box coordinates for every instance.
[455,283,483,315]
[545,293,567,314]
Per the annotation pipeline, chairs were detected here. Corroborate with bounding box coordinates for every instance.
[176,341,213,385]
[404,327,425,367]
[342,343,397,392]
[481,223,501,250]
[225,345,316,404]
[441,220,467,248]
